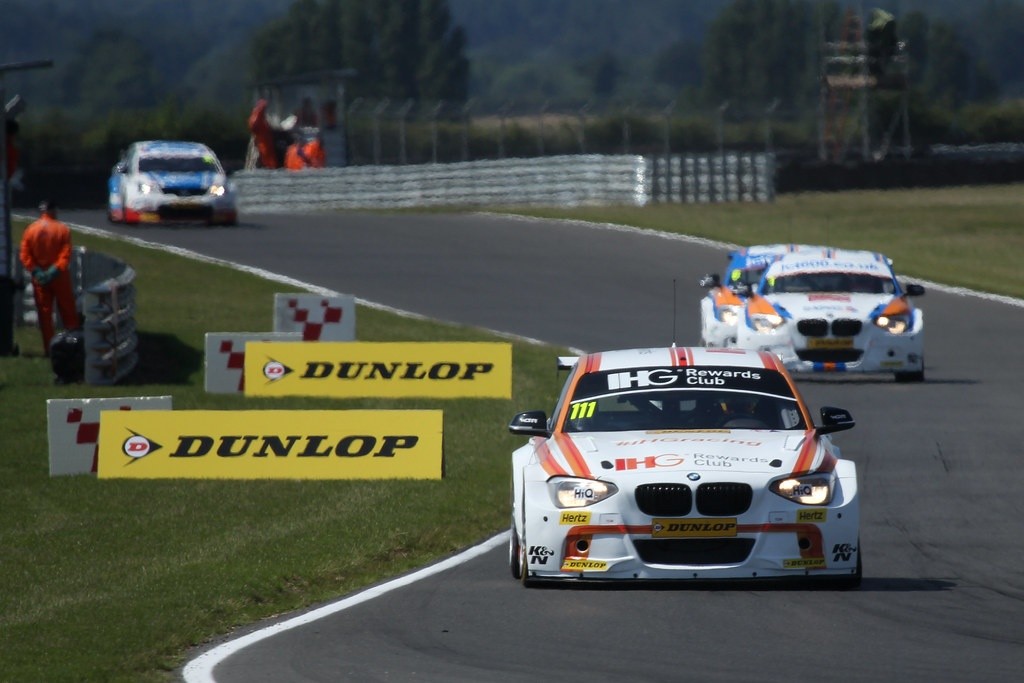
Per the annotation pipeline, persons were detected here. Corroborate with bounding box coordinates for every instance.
[19,200,80,357]
[247,98,326,170]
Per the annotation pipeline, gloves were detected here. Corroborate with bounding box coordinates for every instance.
[32,266,57,285]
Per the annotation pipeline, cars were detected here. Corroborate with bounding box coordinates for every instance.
[109,139,239,228]
[696,245,831,346]
[737,253,928,382]
[510,346,863,588]
